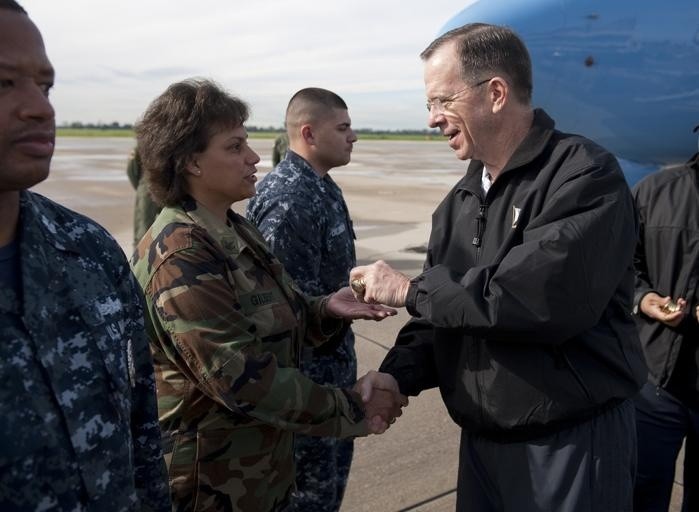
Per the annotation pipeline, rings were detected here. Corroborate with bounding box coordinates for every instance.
[351,277,367,293]
[389,417,395,425]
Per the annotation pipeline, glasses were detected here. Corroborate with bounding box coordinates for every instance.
[425,78,493,112]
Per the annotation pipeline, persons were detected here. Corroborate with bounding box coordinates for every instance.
[126,143,163,251]
[351,24,647,512]
[632,126,699,510]
[130,77,406,511]
[245,87,357,511]
[273,133,289,168]
[0,0,171,512]
